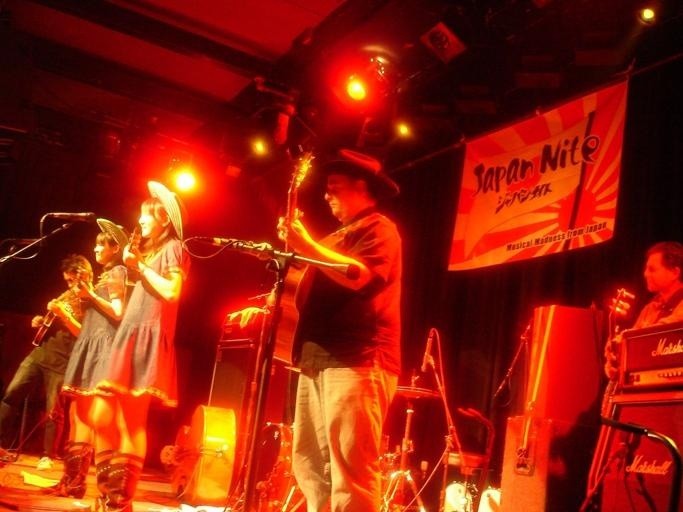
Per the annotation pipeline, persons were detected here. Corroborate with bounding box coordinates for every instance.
[39,219,127,498]
[84,179,187,511]
[0,254,93,470]
[605,240,683,329]
[264,149,404,510]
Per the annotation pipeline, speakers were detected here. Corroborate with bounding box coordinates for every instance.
[600,393,683,512]
[207,309,292,506]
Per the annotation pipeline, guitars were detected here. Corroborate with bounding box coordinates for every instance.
[272,145,318,367]
[123,225,142,311]
[70,261,90,322]
[601,289,635,419]
[32,288,77,347]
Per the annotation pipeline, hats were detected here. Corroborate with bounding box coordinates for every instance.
[96,217,130,255]
[314,148,400,202]
[144,178,186,241]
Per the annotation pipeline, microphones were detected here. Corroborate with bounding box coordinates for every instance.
[421,334,434,372]
[46,212,95,225]
[274,252,360,280]
[6,239,40,245]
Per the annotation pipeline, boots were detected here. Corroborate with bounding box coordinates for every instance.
[91,450,117,511]
[36,441,94,502]
[108,451,148,511]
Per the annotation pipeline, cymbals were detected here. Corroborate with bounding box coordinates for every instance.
[396,386,440,401]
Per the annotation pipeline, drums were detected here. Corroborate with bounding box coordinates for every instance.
[250,422,307,511]
[441,480,500,511]
[178,405,237,504]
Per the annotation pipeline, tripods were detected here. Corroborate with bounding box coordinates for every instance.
[381,392,443,511]
